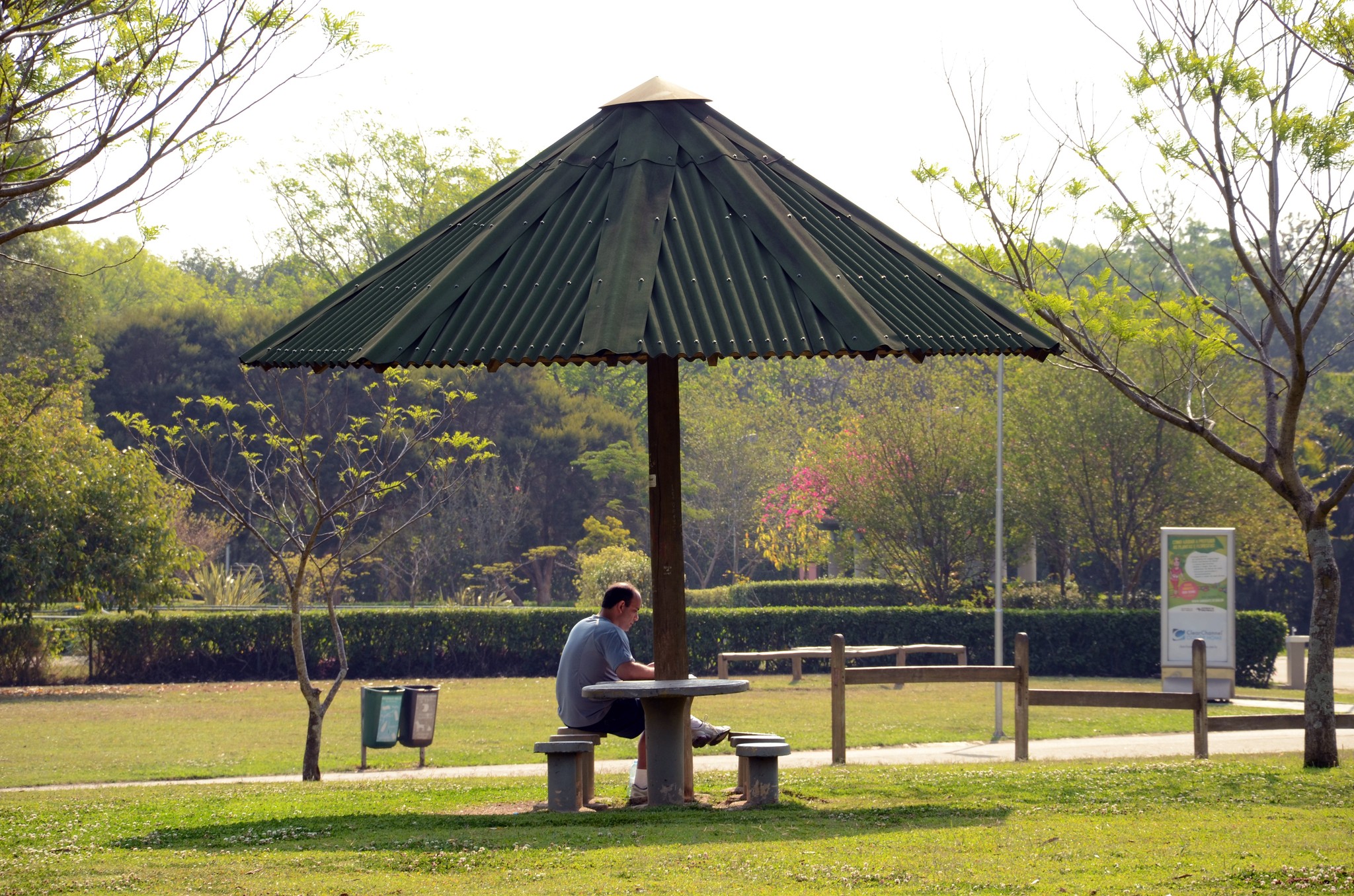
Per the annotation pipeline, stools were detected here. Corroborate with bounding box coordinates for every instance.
[728,731,791,807]
[535,726,607,813]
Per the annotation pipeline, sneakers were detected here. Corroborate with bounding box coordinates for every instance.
[627,783,648,804]
[691,715,730,748]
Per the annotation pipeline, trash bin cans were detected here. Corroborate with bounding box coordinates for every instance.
[364,686,402,748]
[398,686,440,746]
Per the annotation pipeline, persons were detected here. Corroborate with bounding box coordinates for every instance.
[555,582,732,805]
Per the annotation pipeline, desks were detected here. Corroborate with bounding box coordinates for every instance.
[581,676,748,806]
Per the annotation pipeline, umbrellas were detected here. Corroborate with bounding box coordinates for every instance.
[238,76,1066,681]
